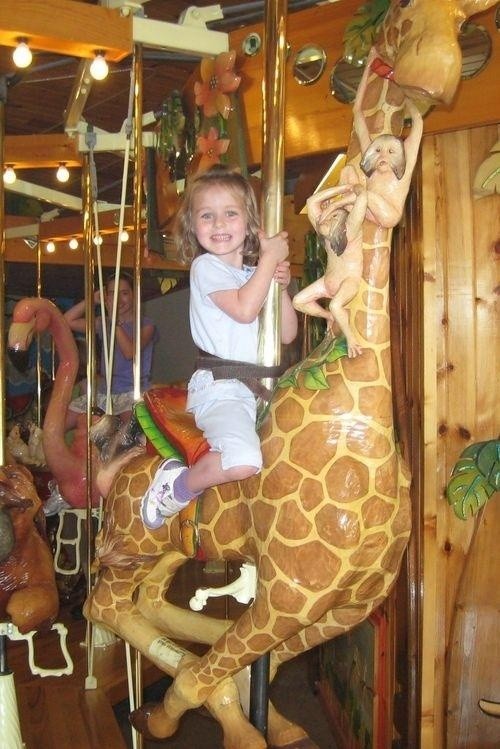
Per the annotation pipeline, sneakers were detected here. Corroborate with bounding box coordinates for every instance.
[140,458,187,530]
[43,487,70,516]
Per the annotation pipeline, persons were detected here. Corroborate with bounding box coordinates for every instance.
[135,164,297,529]
[39,271,160,519]
